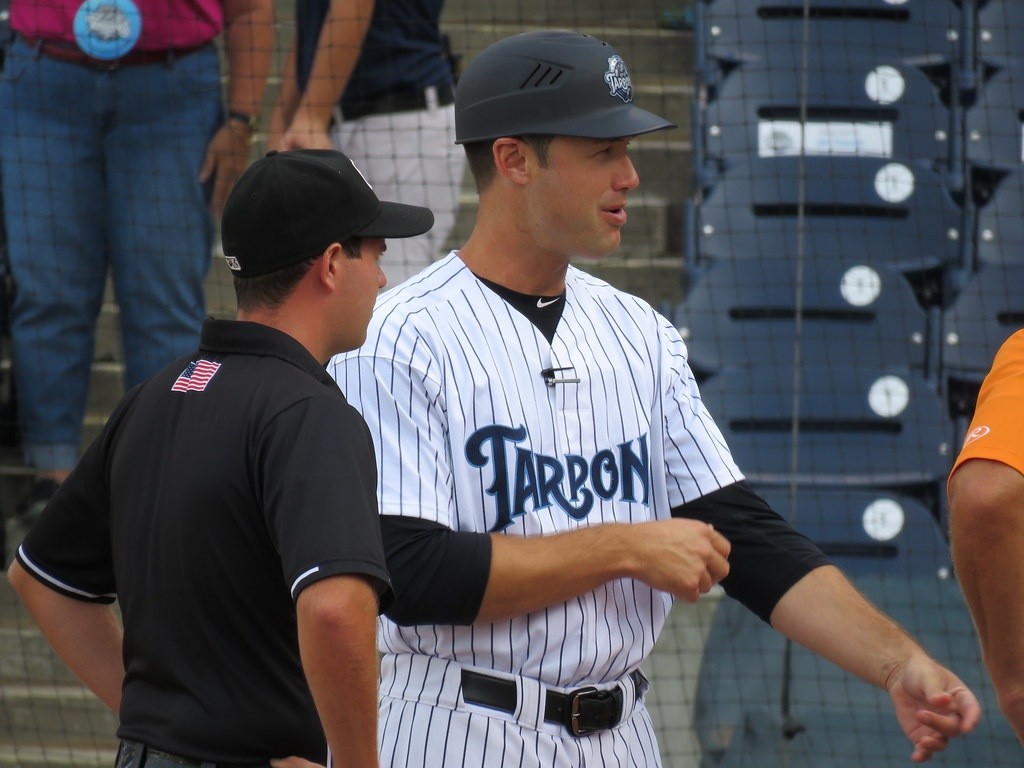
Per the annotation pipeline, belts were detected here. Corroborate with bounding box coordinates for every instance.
[329,81,454,128]
[23,35,207,72]
[458,669,645,736]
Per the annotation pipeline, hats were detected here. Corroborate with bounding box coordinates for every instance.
[220,148,435,278]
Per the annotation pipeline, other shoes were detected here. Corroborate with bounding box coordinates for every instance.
[16,478,59,521]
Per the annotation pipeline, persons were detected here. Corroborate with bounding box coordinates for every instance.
[323,31,983,768]
[949,329,1024,749]
[0,0,470,768]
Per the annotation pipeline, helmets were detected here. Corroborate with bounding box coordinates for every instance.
[455,29,677,144]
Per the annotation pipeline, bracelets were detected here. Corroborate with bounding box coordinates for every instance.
[227,106,261,135]
